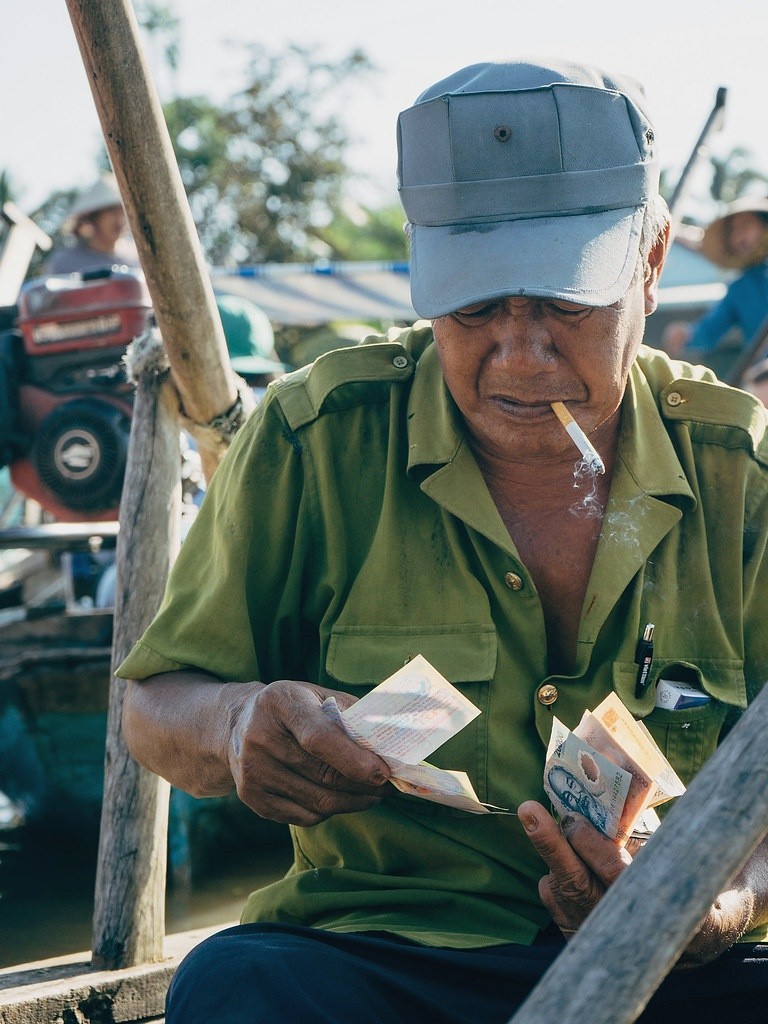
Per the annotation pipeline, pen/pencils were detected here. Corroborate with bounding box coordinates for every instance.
[632,620,655,700]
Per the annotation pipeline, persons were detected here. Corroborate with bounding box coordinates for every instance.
[681,188,768,369]
[112,52,767,1024]
[28,179,136,280]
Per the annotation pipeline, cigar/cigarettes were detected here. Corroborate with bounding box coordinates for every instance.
[550,401,606,475]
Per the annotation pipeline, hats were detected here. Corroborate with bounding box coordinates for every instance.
[62,177,125,236]
[210,292,283,377]
[396,59,659,322]
[699,188,768,269]
